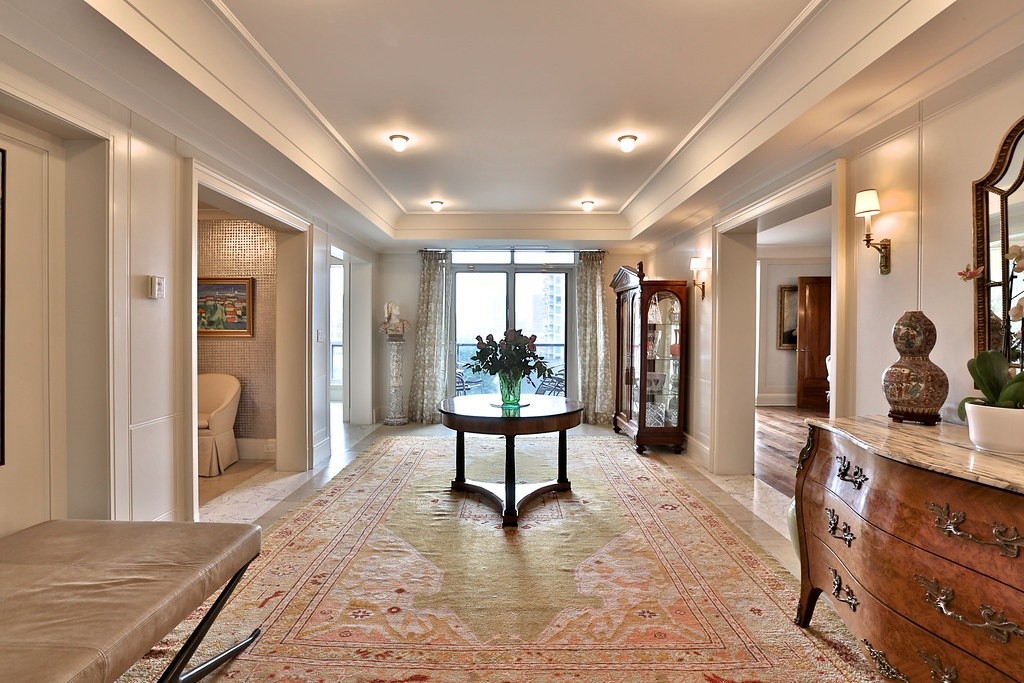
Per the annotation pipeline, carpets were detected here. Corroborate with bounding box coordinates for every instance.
[113,435,903,683]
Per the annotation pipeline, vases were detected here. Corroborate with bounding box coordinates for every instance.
[963,401,1024,454]
[498,367,522,408]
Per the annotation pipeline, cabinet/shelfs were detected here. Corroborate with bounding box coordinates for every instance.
[609,259,689,454]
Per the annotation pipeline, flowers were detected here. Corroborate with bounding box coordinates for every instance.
[462,329,555,398]
[958,245,1024,421]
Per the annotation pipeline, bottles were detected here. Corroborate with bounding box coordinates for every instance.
[882,311,949,415]
[648,293,663,324]
[668,299,680,324]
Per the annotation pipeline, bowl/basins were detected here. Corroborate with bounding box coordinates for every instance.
[646,372,666,391]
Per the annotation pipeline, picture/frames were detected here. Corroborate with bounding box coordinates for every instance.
[197,277,256,340]
[776,285,798,350]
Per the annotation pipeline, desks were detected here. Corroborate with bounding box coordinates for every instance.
[795,413,1024,683]
[438,393,585,526]
[0,520,261,683]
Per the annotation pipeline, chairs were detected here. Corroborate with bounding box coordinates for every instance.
[455,369,483,395]
[534,364,566,397]
[197,374,242,477]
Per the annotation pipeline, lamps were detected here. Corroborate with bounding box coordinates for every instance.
[431,201,444,210]
[617,134,636,151]
[581,199,595,211]
[855,188,891,275]
[689,257,705,299]
[389,134,410,151]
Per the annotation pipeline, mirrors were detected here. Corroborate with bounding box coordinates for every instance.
[972,116,1024,390]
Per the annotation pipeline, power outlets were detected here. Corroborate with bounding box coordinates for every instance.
[263,445,275,453]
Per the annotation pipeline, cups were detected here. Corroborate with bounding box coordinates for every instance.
[646,402,665,427]
[647,330,662,358]
